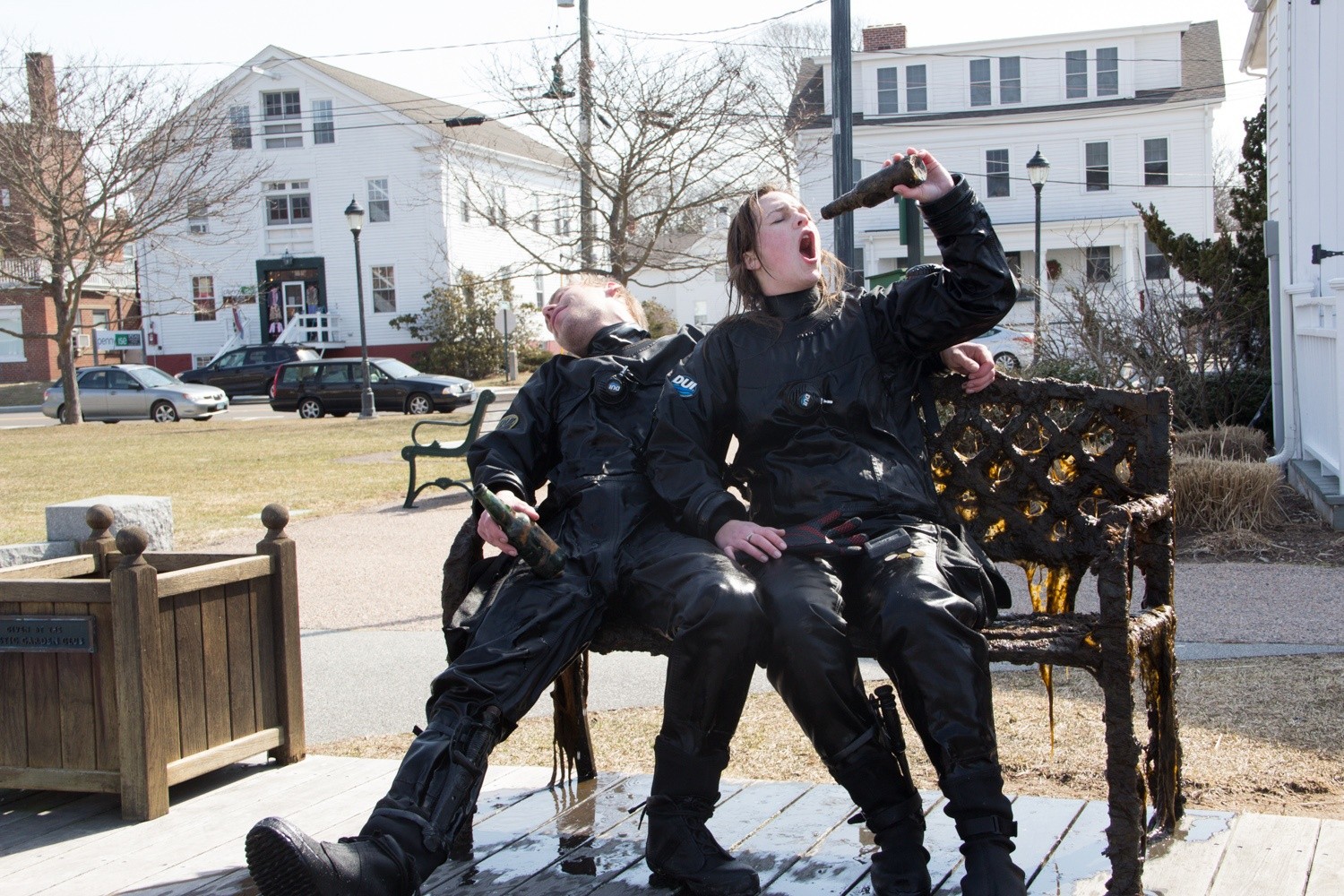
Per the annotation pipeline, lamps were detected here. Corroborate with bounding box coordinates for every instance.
[283,250,293,266]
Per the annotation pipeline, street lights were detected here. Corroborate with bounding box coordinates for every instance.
[1026,145,1049,362]
[345,198,378,418]
[543,35,592,270]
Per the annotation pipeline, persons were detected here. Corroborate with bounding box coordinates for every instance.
[678,147,1021,896]
[245,281,995,896]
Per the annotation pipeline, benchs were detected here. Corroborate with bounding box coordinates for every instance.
[402,391,522,509]
[549,362,1186,896]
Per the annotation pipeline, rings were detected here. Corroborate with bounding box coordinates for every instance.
[747,532,753,542]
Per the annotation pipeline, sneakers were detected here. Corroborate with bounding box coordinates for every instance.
[244,816,420,896]
[627,792,761,896]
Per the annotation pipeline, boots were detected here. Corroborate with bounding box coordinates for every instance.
[941,772,1029,896]
[848,787,931,896]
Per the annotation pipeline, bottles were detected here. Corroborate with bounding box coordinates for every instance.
[472,483,569,579]
[821,154,928,219]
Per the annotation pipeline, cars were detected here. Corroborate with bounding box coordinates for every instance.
[270,357,478,419]
[969,326,1035,372]
[174,342,323,400]
[42,363,229,424]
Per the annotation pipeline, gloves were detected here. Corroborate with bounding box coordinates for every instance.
[735,509,868,563]
